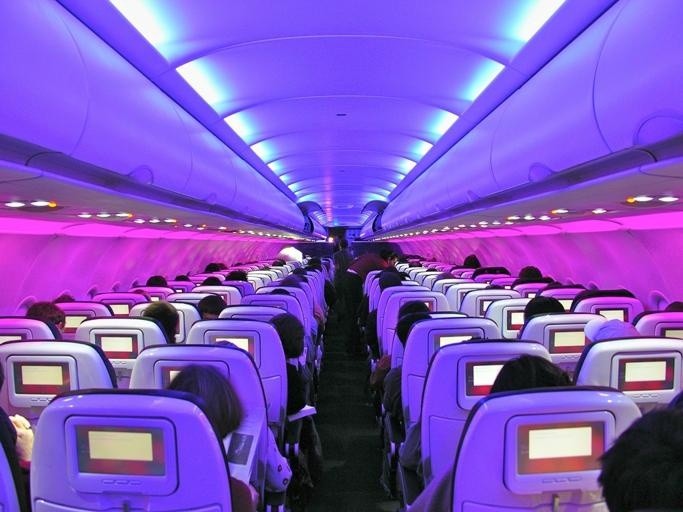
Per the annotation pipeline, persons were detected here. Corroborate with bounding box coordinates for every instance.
[1,236,683,512]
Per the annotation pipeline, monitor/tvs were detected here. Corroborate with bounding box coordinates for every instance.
[65,415,178,495]
[6,355,77,407]
[610,350,681,405]
[504,411,616,495]
[61,285,287,363]
[1,328,33,346]
[399,279,633,355]
[457,356,515,411]
[655,322,683,339]
[153,360,230,391]
